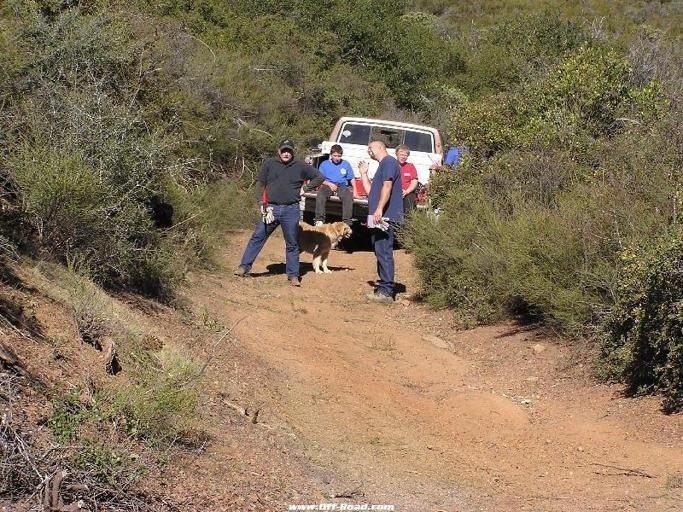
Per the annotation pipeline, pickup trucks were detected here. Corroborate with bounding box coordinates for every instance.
[299,116,444,232]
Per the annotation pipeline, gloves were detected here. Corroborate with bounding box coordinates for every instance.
[260,204,275,225]
[367,215,390,232]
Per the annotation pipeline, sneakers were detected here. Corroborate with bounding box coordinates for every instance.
[364,291,395,304]
[289,274,301,286]
[233,265,247,276]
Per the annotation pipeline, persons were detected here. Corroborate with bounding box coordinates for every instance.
[393,144,419,248]
[314,143,362,229]
[355,140,404,304]
[434,146,458,171]
[233,138,326,287]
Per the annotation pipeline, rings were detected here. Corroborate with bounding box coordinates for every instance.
[330,187,332,189]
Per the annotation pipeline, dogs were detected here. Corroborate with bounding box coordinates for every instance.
[298,220,353,274]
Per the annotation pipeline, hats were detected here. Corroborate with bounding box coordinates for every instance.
[279,139,295,152]
